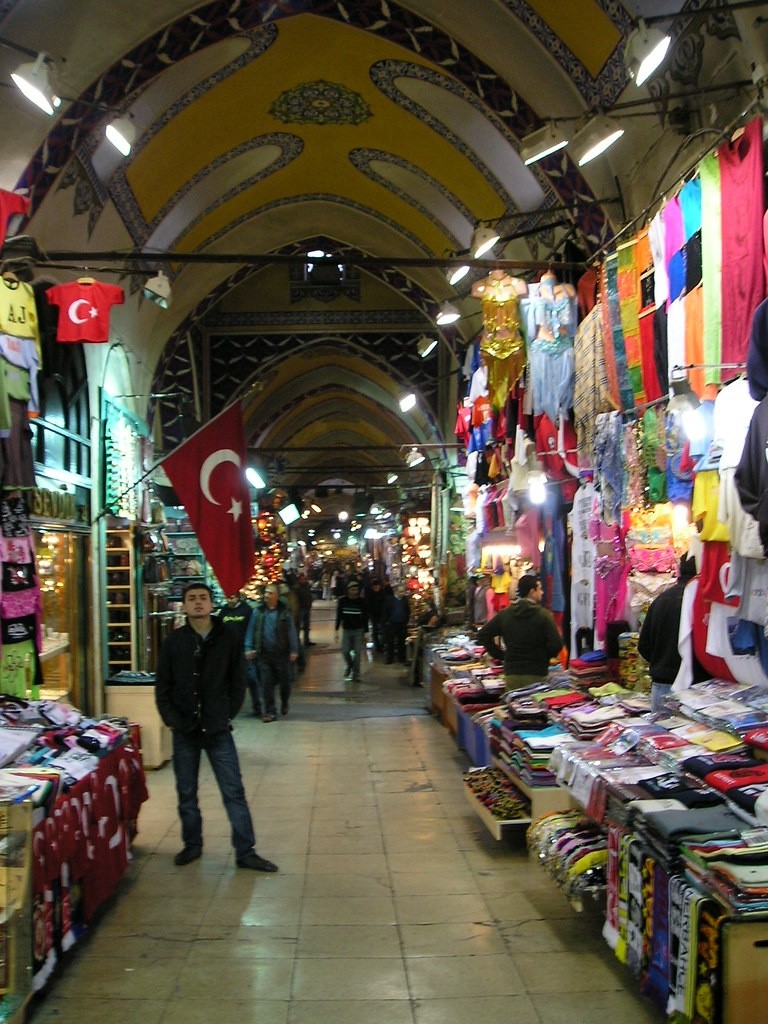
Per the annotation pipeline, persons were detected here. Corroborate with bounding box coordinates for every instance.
[471,261,605,429]
[589,483,628,640]
[243,583,300,723]
[512,470,551,574]
[155,582,279,873]
[285,567,440,682]
[217,591,263,719]
[476,575,564,693]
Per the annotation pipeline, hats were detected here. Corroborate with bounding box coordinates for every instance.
[347,580,359,589]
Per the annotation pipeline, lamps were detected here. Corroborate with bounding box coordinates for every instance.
[33,262,174,309]
[417,312,482,357]
[370,497,415,520]
[386,456,439,484]
[527,452,546,505]
[0,82,137,157]
[0,37,69,116]
[521,105,685,166]
[665,362,747,414]
[304,497,323,513]
[623,0,768,87]
[565,79,753,167]
[397,368,461,413]
[441,218,574,285]
[469,196,619,259]
[400,443,466,468]
[451,500,465,510]
[435,270,531,325]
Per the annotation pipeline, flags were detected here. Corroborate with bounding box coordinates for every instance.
[160,397,258,599]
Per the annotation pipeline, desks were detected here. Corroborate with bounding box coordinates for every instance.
[0,704,149,996]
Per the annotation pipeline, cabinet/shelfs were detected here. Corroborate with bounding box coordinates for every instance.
[106,522,138,673]
[104,685,173,769]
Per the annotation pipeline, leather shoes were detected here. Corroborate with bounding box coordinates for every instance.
[174,847,202,865]
[236,854,278,871]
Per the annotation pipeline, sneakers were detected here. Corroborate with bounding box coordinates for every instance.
[281,702,288,715]
[263,713,277,722]
[252,709,262,716]
[344,666,351,676]
[353,674,360,681]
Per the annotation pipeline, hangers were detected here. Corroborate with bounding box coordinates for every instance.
[581,476,587,489]
[1,269,19,283]
[74,267,95,285]
